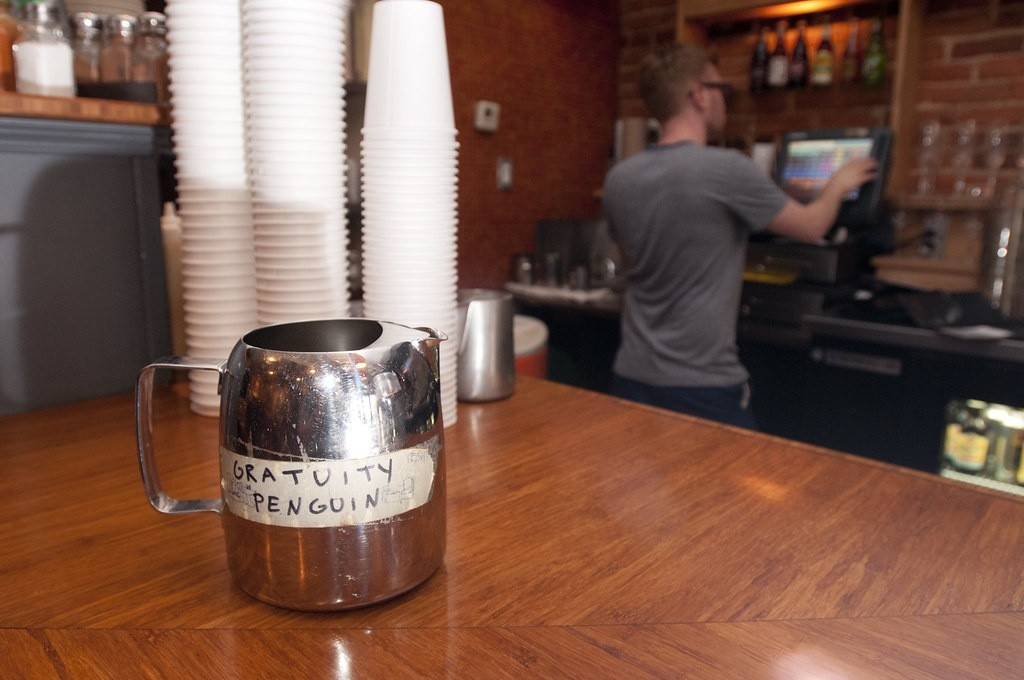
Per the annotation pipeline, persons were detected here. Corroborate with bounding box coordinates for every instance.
[603,45,879,422]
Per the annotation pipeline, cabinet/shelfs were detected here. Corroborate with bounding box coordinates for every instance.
[0,90,162,417]
[676,0,926,197]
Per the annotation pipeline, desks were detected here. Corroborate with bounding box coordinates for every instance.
[0,373,1024,680]
[506,279,1024,475]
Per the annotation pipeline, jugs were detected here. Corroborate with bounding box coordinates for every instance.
[134,317,447,612]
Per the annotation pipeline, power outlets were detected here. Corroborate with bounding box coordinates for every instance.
[496,157,513,191]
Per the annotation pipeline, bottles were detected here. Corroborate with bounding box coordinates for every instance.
[751,14,888,96]
[159,201,186,358]
[11,0,170,103]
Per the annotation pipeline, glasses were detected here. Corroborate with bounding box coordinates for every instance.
[688,82,736,102]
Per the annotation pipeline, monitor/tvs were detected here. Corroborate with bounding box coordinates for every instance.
[771,127,891,225]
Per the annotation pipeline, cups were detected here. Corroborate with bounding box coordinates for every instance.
[155,0,459,430]
[457,287,516,403]
